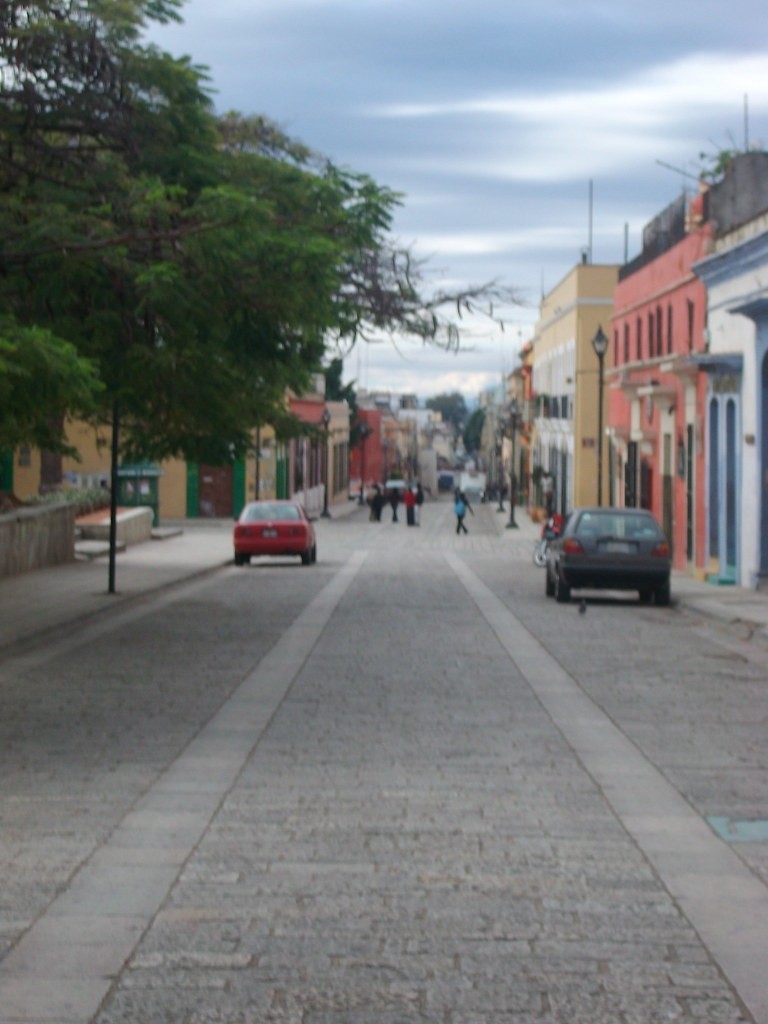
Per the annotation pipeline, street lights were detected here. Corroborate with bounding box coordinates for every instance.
[381,435,389,481]
[321,407,334,517]
[356,420,368,504]
[506,399,520,528]
[591,325,610,507]
[497,437,506,514]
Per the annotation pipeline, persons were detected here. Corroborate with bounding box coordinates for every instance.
[454,487,474,535]
[366,482,424,526]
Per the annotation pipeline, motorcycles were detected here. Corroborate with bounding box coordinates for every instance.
[533,512,562,568]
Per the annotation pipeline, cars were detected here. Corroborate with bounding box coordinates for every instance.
[232,500,319,565]
[385,478,425,504]
[545,507,670,607]
